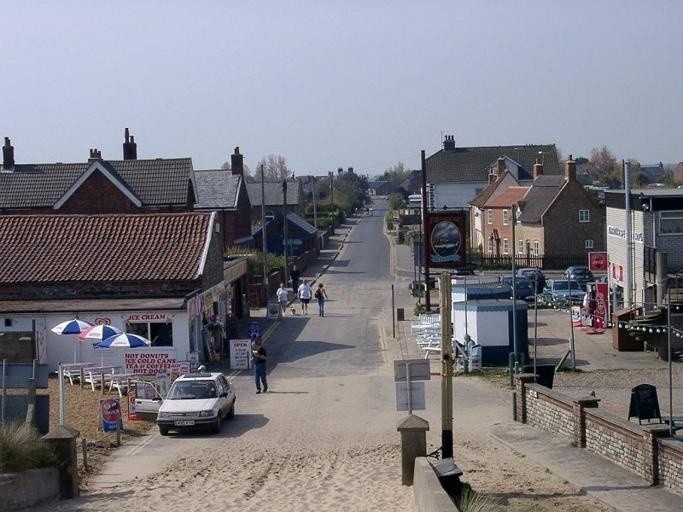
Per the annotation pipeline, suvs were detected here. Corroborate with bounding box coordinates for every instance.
[543,279,586,303]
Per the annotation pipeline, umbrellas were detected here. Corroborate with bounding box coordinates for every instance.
[49,319,92,364]
[93,330,152,374]
[75,324,123,367]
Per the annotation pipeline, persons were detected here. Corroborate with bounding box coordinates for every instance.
[317,283,327,316]
[275,281,290,316]
[289,264,299,296]
[462,335,474,356]
[296,278,312,316]
[249,339,267,394]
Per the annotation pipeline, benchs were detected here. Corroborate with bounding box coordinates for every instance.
[416,331,442,359]
[54,370,141,397]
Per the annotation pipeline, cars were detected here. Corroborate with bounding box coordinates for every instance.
[503,267,545,298]
[134,372,236,436]
[564,266,594,288]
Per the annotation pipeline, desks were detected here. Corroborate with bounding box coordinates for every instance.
[411,324,439,330]
[60,363,121,374]
